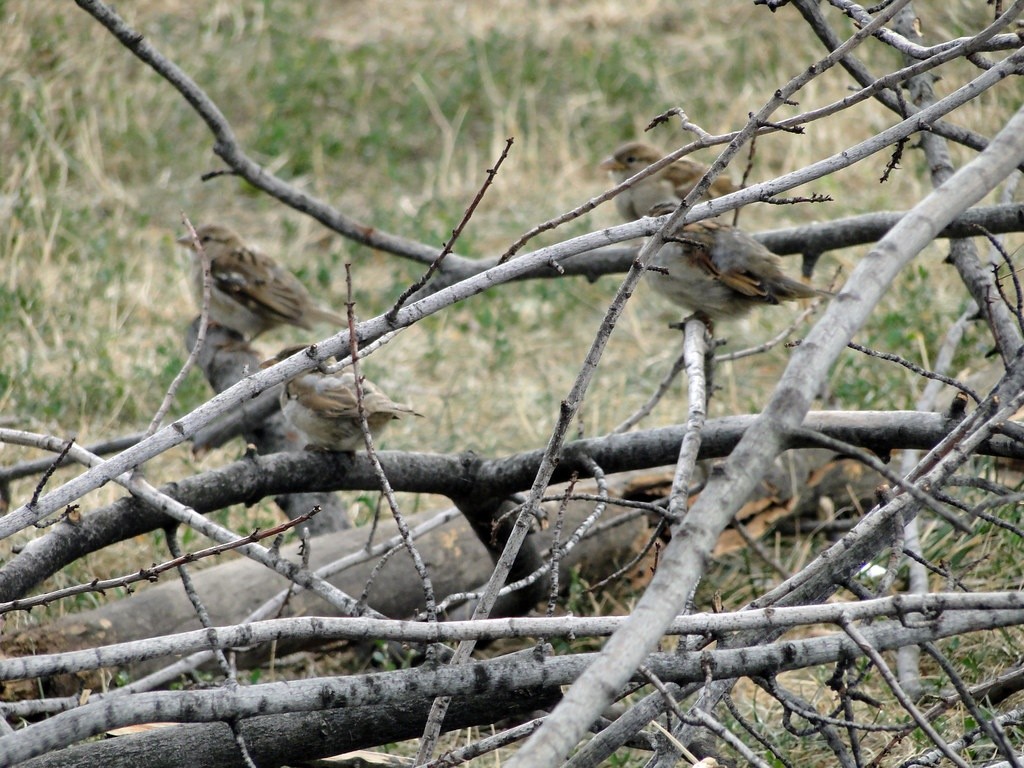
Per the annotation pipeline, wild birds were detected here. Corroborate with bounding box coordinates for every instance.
[596,140,822,342]
[256,342,428,468]
[174,221,351,358]
